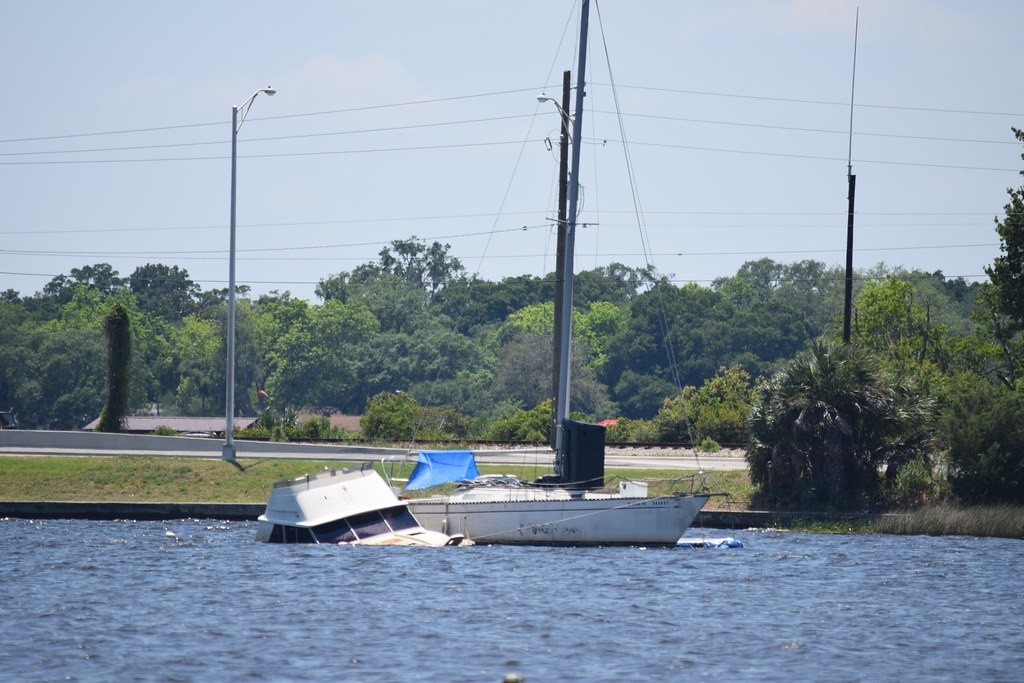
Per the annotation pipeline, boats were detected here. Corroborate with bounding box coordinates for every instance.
[254,465,476,548]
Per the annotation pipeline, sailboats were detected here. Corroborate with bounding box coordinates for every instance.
[402,2,712,551]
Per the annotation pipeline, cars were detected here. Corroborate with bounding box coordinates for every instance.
[0,412,19,430]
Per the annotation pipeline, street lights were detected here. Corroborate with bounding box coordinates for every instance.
[223,88,276,458]
[535,94,584,469]
